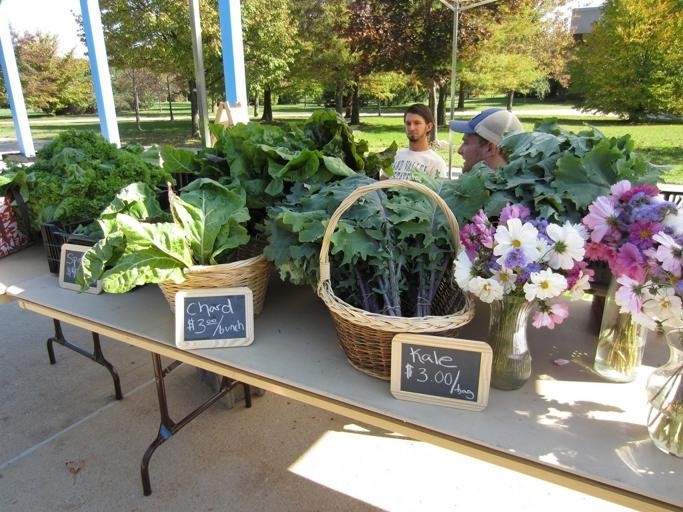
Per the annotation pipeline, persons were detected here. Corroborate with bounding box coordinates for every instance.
[378,102,447,183]
[445,106,524,175]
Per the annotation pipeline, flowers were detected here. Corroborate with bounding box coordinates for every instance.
[454,201,592,330]
[580,181,681,330]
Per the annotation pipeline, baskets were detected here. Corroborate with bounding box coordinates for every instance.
[316,180,477,380]
[157,238,270,315]
[40,218,140,292]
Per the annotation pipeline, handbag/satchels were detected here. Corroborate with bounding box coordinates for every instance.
[0,183,35,259]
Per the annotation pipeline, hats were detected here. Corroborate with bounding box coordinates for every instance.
[448,109,524,145]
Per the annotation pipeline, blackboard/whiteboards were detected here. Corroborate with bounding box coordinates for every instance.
[390,333,494,412]
[175,286,254,351]
[58,243,104,294]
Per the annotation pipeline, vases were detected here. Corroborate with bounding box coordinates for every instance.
[639,332,683,461]
[480,295,535,393]
[593,267,651,386]
[481,272,683,460]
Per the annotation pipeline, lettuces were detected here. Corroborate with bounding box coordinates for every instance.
[0,129,177,233]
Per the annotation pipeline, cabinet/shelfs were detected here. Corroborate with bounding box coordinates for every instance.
[0,238,683,512]
